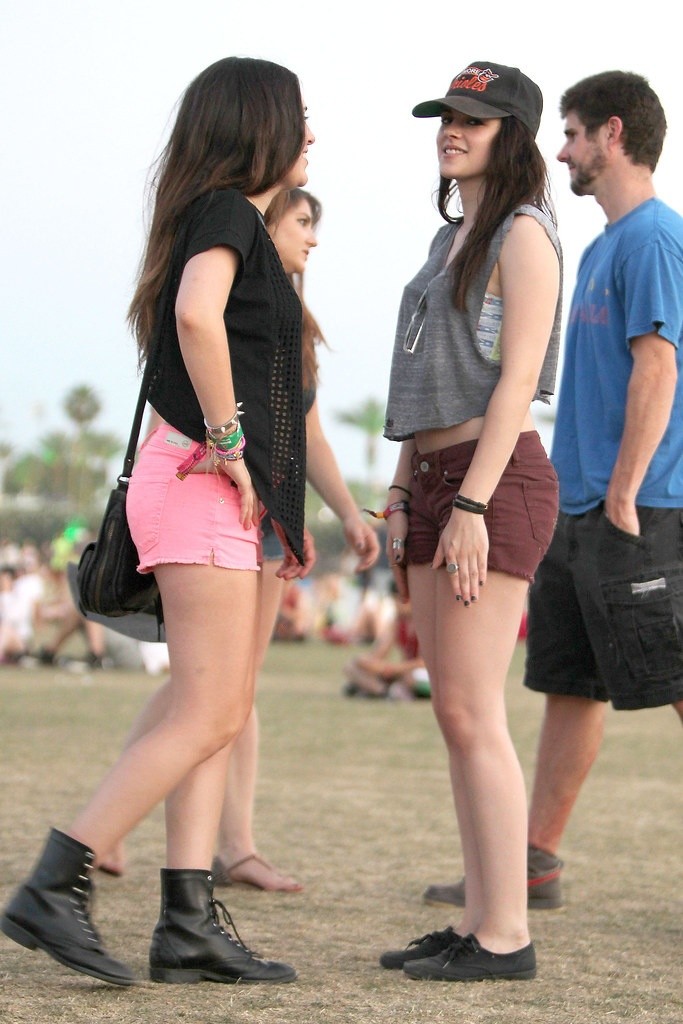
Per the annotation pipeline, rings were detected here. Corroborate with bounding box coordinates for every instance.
[445,563,459,574]
[392,538,404,550]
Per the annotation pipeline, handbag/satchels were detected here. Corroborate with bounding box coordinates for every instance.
[76,482,167,643]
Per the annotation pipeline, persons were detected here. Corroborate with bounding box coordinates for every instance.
[377,61,563,981]
[420,69,683,910]
[0,510,391,673]
[0,56,317,987]
[86,188,379,892]
[343,580,434,702]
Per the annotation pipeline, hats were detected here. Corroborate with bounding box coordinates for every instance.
[412,61,543,137]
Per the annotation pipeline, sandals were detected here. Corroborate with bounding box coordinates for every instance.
[99,839,126,877]
[212,855,305,893]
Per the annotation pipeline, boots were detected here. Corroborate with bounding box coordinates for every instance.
[0,827,134,985]
[150,868,298,984]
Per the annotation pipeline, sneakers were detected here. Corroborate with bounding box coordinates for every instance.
[379,926,466,970]
[422,843,563,916]
[403,934,537,982]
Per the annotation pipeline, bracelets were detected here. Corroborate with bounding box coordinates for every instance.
[452,493,488,515]
[389,485,412,497]
[361,500,409,521]
[195,402,246,505]
